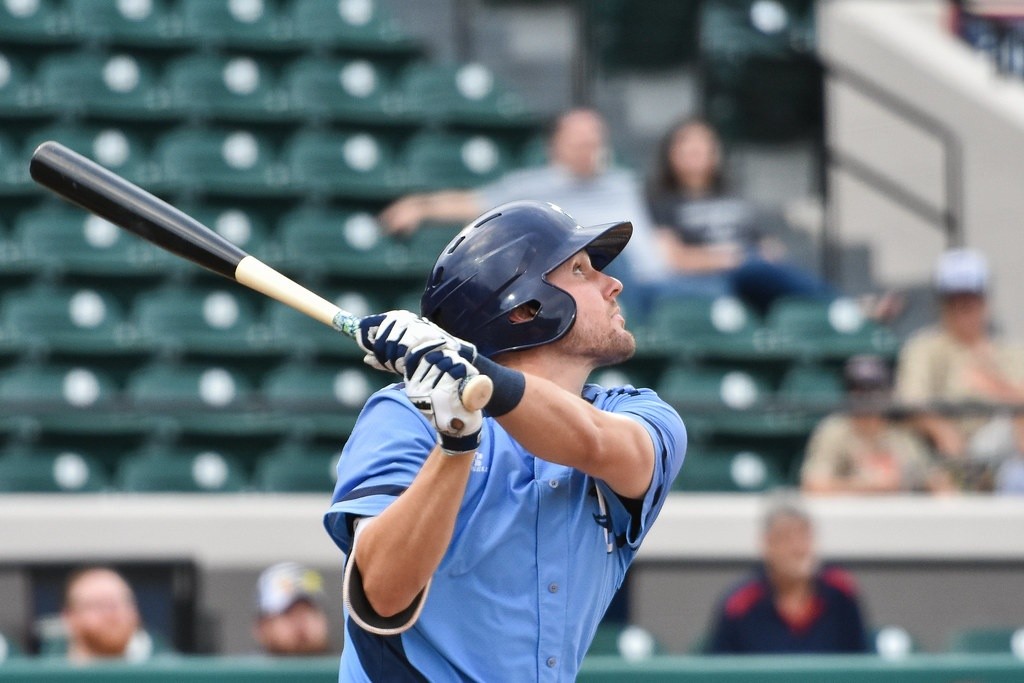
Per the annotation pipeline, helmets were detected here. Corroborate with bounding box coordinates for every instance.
[420,200,634,359]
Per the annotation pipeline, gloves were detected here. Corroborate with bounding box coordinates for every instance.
[399,338,492,456]
[356,309,478,400]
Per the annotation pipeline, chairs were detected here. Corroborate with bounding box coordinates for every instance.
[0,0,901,491]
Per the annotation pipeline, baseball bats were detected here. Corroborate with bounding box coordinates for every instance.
[28,141,495,415]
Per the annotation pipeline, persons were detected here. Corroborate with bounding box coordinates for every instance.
[696,507,872,655]
[64,565,159,666]
[798,251,1024,494]
[378,110,723,319]
[322,203,688,683]
[654,118,908,326]
[248,557,328,657]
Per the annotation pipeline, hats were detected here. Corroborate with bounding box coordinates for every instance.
[934,248,989,297]
[256,561,328,618]
